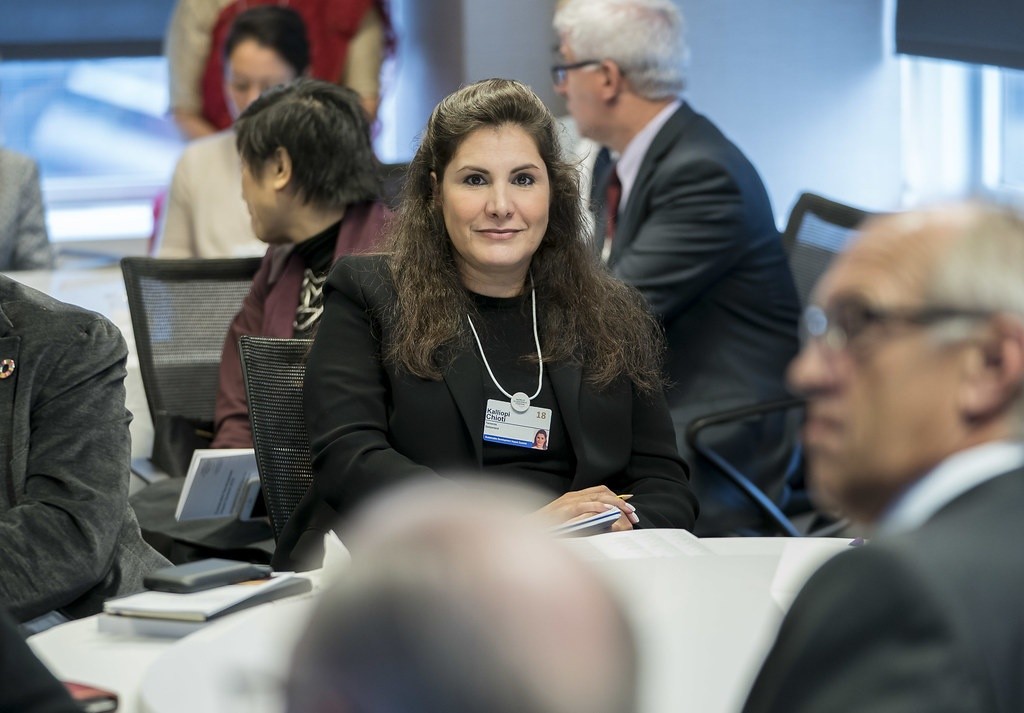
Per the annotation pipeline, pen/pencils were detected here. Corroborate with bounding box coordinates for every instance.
[617,494,634,501]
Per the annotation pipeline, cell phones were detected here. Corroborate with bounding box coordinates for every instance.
[236,477,268,522]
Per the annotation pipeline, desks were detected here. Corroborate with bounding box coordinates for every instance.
[25,529,867,713]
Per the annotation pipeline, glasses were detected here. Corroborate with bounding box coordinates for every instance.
[795,292,994,360]
[551,60,599,87]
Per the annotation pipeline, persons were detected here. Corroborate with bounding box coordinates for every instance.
[533,430,548,448]
[289,78,701,532]
[285,512,642,713]
[1,0,821,713]
[732,202,1023,712]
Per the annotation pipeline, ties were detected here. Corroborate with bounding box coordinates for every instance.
[602,168,622,239]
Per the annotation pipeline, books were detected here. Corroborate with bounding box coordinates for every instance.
[102,568,292,621]
[55,679,118,711]
[551,509,624,537]
[209,578,310,623]
[173,447,300,523]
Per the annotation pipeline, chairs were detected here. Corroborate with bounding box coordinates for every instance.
[116,254,268,479]
[236,330,320,528]
[782,192,901,538]
